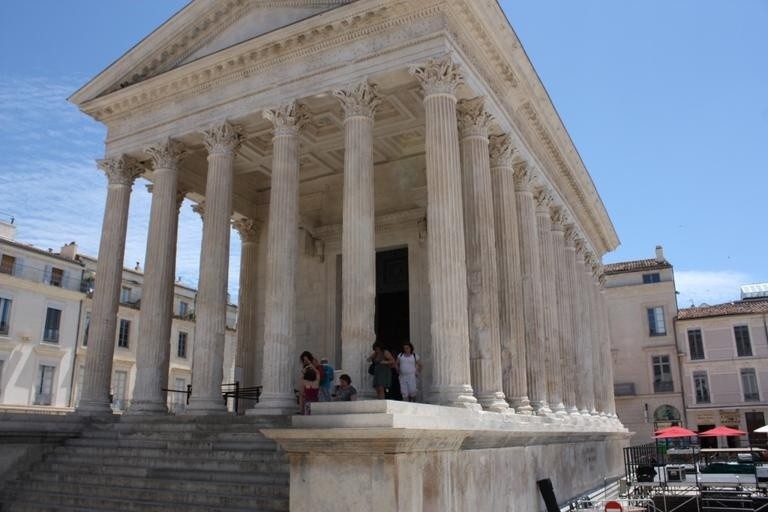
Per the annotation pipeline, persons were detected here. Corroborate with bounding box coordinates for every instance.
[293,338,358,416]
[365,341,397,399]
[394,341,424,403]
[637,450,658,481]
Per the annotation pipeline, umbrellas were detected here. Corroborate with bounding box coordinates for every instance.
[650,423,768,448]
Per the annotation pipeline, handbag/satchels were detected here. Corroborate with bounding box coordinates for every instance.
[369,359,375,374]
[302,366,316,381]
